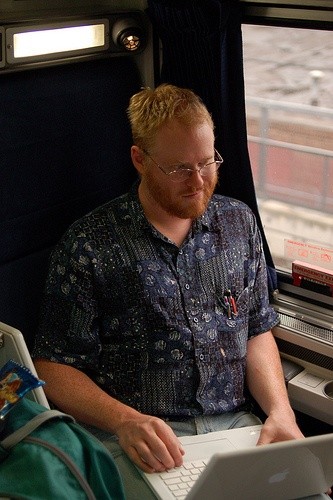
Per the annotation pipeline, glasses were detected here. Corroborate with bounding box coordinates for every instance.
[143,149,224,183]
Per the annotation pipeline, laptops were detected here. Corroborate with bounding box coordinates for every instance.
[134,421,333,500]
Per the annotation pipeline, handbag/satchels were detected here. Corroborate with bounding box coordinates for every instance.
[1,395,126,500]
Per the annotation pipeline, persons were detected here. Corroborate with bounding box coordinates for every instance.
[24,84,333,500]
